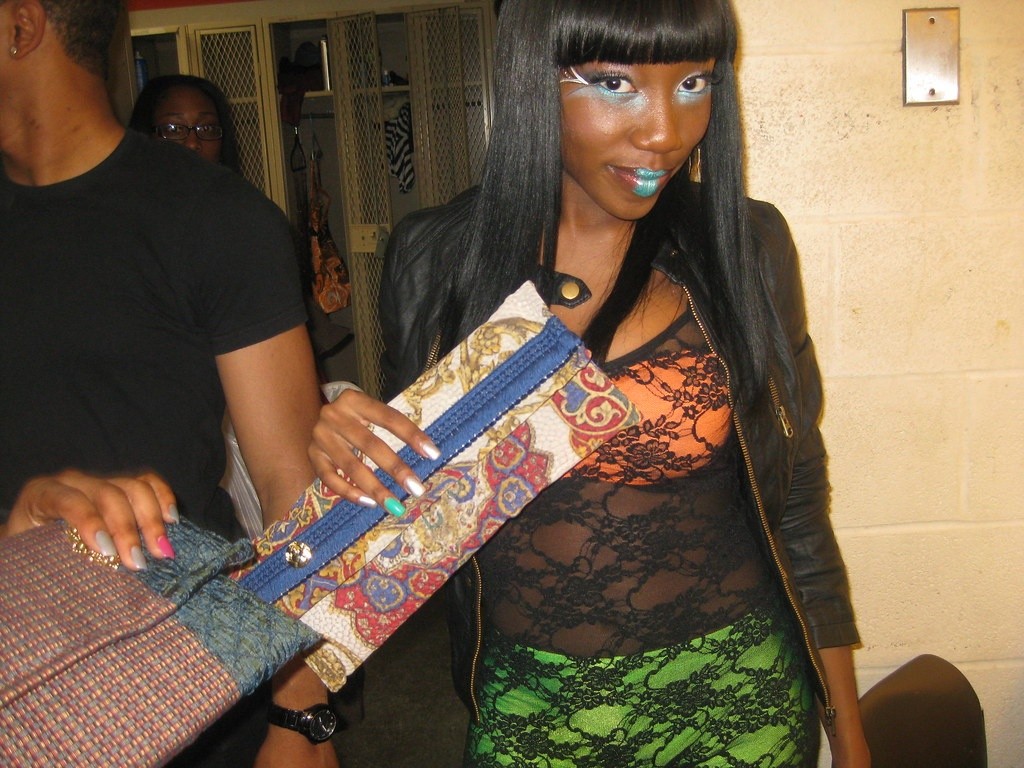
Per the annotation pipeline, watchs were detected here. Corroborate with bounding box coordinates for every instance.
[268,699,337,745]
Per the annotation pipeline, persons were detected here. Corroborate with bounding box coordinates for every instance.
[125,75,243,176]
[0,1,338,768]
[307,0,871,768]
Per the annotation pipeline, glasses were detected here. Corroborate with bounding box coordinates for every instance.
[149,123,223,140]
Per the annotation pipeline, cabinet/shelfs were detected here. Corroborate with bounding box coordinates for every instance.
[122,0,500,403]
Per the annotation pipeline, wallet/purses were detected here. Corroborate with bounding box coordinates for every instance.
[230,280,648,695]
